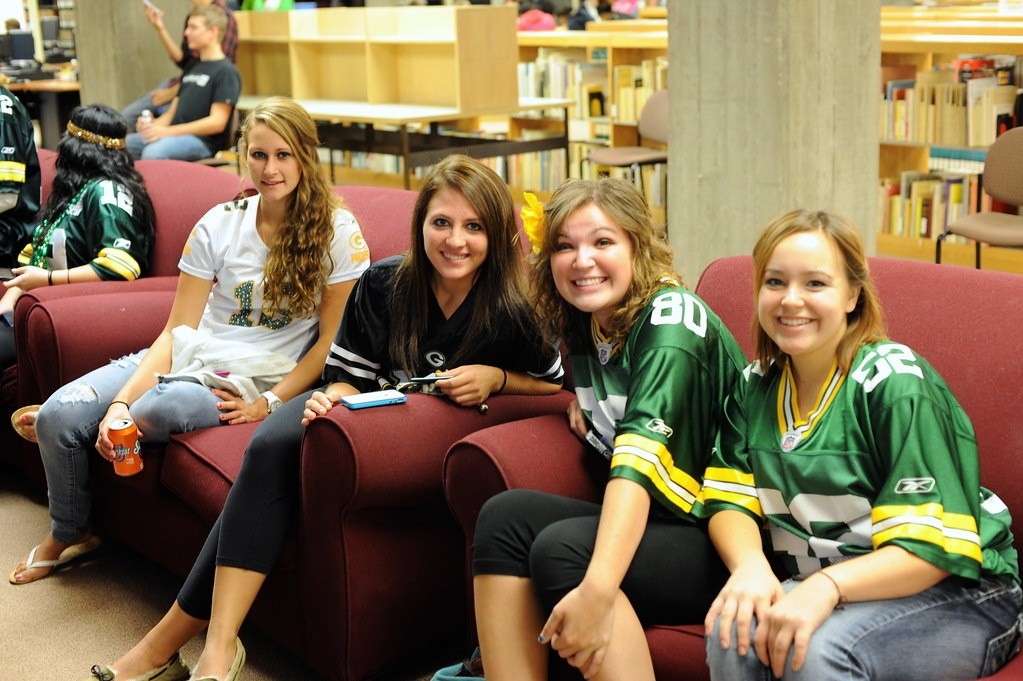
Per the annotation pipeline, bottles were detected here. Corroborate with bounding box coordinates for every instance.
[141,109,154,126]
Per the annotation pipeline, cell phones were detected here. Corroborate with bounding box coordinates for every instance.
[340,389,408,409]
[0,268,18,280]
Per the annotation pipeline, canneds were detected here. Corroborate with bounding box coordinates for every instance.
[107,419,144,476]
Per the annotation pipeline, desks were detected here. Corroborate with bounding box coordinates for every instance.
[236,96,577,190]
[3,78,80,154]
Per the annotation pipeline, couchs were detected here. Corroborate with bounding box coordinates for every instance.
[30,188,580,680]
[1,148,253,480]
[439,255,1023,681]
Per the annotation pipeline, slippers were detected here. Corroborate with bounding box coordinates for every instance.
[10,405,37,443]
[9,536,100,584]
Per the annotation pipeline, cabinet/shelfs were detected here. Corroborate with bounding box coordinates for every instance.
[502,37,676,238]
[881,51,1022,260]
[232,6,576,109]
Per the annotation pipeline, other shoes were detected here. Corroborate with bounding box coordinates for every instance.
[188,637,246,681]
[83,650,189,681]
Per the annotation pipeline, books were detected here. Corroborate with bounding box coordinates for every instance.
[312,47,668,205]
[881,69,1023,244]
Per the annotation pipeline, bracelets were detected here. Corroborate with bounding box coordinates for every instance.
[67,269,70,283]
[499,368,507,390]
[48,268,53,285]
[816,569,847,610]
[106,401,129,408]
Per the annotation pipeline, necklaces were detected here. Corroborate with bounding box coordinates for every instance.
[32,179,93,270]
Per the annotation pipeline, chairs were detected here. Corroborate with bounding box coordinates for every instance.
[935,126,1023,271]
[190,109,242,170]
[579,91,672,234]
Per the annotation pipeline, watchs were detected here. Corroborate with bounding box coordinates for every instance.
[262,391,284,413]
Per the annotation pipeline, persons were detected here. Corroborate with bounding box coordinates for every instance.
[118,0,238,135]
[0,102,156,316]
[10,96,371,584]
[516,0,637,35]
[124,4,241,159]
[89,154,564,681]
[702,207,1023,681]
[471,178,746,681]
[0,86,42,268]
